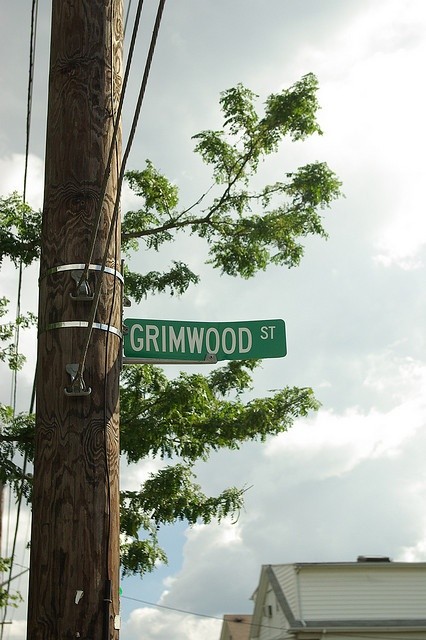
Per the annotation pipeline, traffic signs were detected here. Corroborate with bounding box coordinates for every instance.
[128,317,288,360]
